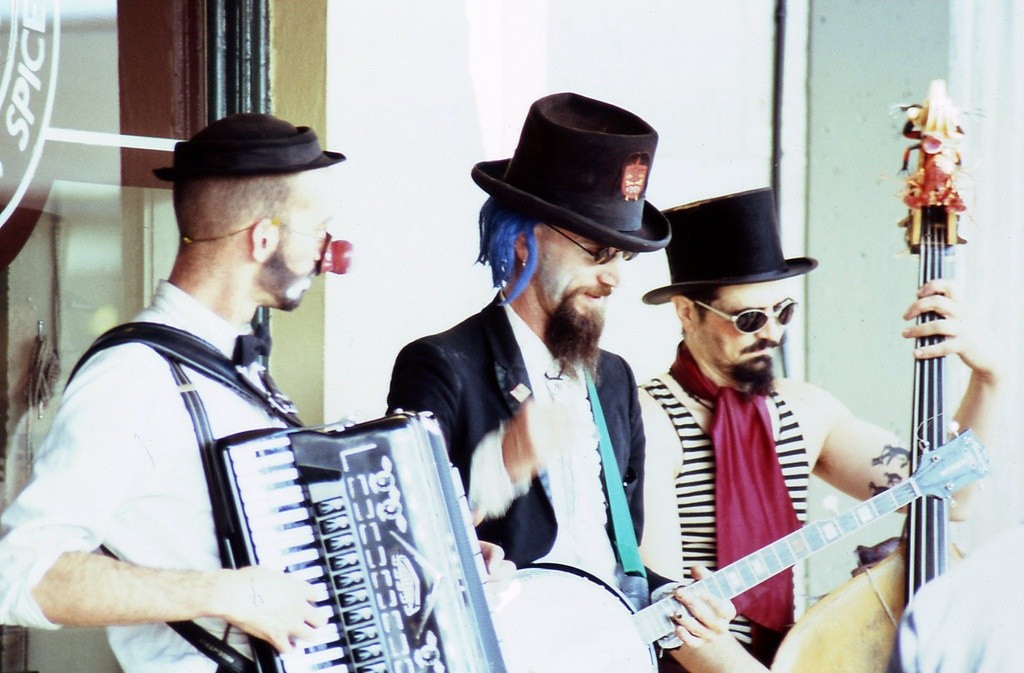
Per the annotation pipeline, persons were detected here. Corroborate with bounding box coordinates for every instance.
[0,111,517,673]
[385,91,737,673]
[636,184,1008,673]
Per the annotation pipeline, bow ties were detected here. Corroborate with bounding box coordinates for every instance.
[232,323,273,367]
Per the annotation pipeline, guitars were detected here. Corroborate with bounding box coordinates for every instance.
[499,420,995,673]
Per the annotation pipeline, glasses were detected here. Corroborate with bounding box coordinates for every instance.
[544,223,641,264]
[694,300,799,334]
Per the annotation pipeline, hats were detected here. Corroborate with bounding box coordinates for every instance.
[153,113,348,181]
[471,92,672,252]
[642,188,819,304]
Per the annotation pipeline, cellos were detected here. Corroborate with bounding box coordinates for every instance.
[758,69,1024,673]
